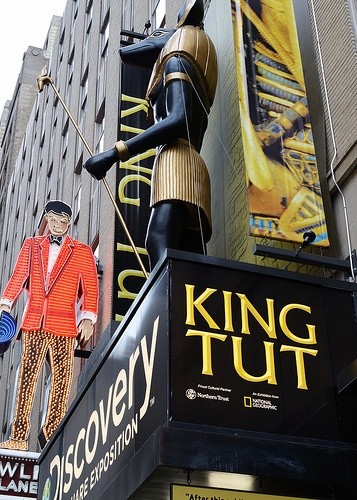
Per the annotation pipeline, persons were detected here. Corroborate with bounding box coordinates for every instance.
[0,200,100,451]
[86,1,217,271]
